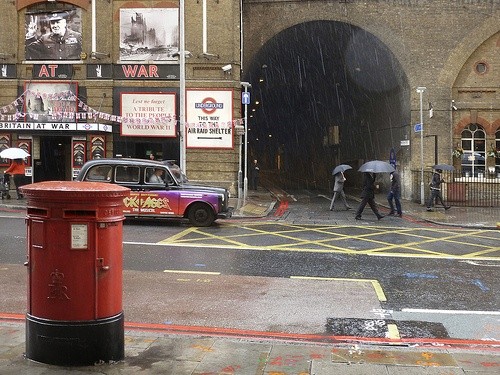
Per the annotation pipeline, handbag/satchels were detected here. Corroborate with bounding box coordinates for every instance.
[429,182,433,187]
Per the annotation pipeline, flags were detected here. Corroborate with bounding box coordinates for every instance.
[0,89,244,129]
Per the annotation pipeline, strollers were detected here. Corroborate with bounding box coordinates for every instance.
[0,172,11,200]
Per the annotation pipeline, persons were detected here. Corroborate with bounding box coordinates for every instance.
[4,158,25,200]
[248,160,261,191]
[149,168,164,183]
[386,172,402,217]
[355,172,385,220]
[25,11,82,59]
[329,172,353,211]
[149,152,158,161]
[426,169,452,212]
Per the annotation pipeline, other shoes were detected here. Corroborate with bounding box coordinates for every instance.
[445,206,451,210]
[427,207,434,212]
[346,207,353,210]
[395,211,402,217]
[388,212,395,215]
[355,216,364,221]
[378,215,385,220]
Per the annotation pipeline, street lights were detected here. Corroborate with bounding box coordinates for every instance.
[415,86,425,204]
[241,82,252,199]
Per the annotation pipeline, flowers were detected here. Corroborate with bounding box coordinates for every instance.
[487,147,500,158]
[452,147,464,159]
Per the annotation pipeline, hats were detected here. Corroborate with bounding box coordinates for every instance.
[47,11,69,21]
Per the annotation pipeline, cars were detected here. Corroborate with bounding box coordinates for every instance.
[76,158,229,229]
[462,149,484,164]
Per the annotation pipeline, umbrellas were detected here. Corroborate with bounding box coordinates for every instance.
[0,148,30,159]
[332,165,352,179]
[358,160,396,185]
[432,164,456,179]
